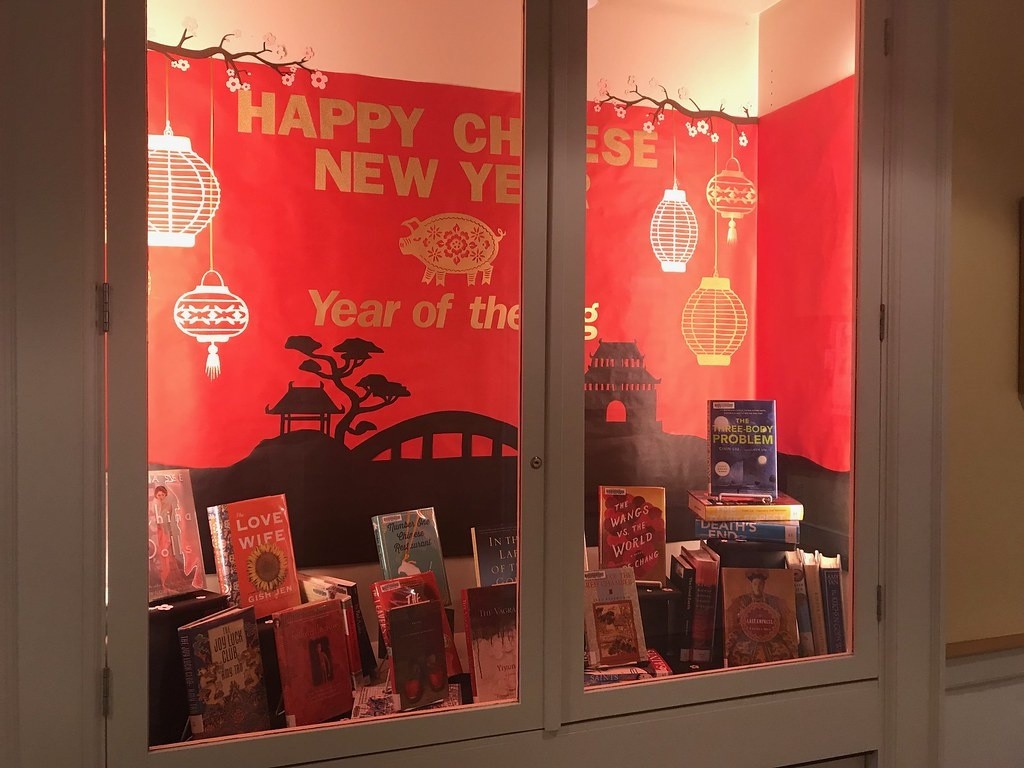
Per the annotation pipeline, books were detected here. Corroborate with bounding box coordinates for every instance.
[147,468,207,599]
[599,486,666,587]
[178,494,464,740]
[466,583,518,702]
[708,400,777,502]
[471,523,518,586]
[584,566,648,668]
[584,489,847,688]
